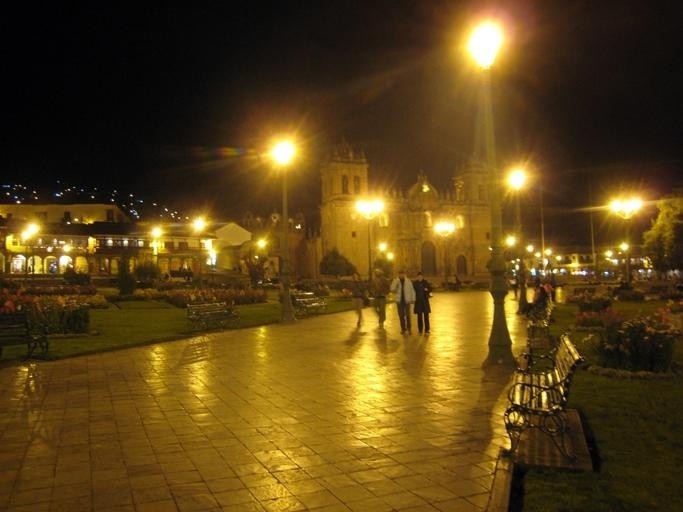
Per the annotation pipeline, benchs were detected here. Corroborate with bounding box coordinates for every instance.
[503,330,587,463]
[1,308,51,362]
[183,298,240,332]
[525,290,556,352]
[290,289,328,319]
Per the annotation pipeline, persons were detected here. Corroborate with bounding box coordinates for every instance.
[452,272,462,292]
[387,270,417,335]
[530,272,556,303]
[315,279,329,296]
[350,270,368,329]
[409,270,432,336]
[370,268,391,329]
[512,274,520,299]
